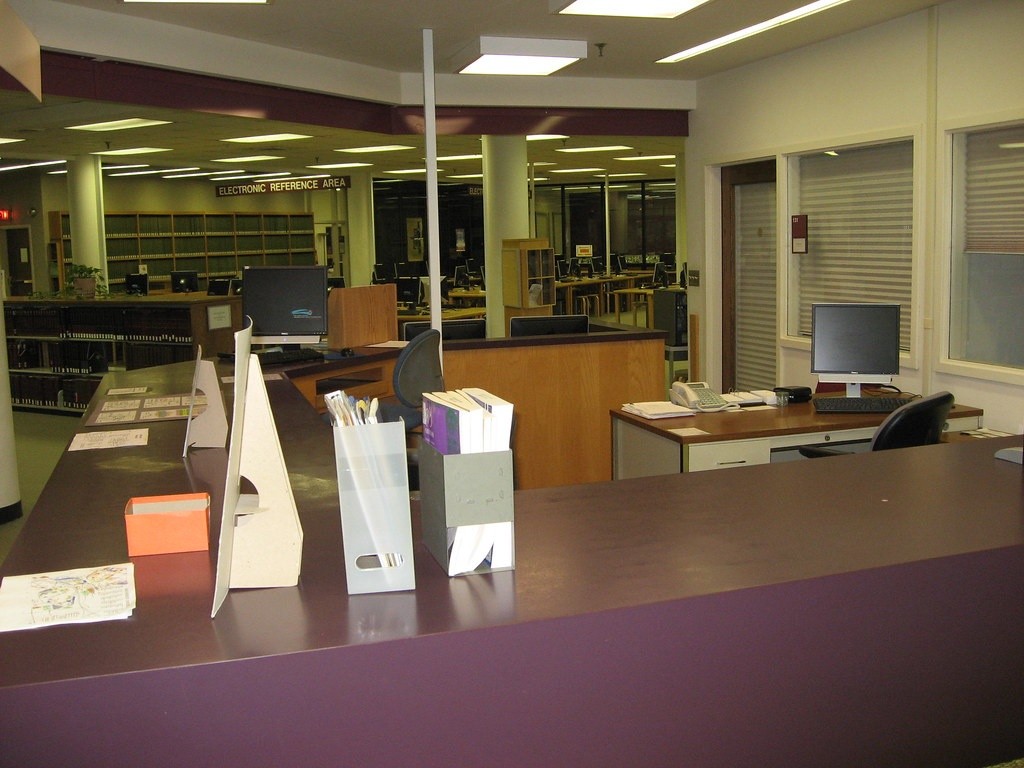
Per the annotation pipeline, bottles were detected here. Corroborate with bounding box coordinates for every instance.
[662,271,668,288]
[680,262,687,287]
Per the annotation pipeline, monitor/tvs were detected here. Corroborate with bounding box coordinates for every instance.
[327,277,345,288]
[403,318,486,340]
[653,252,676,288]
[609,254,627,276]
[208,279,243,296]
[557,254,581,279]
[127,273,149,296]
[171,270,199,294]
[510,315,589,337]
[811,303,900,398]
[454,265,470,291]
[374,261,449,310]
[466,259,482,274]
[241,265,329,351]
[680,262,688,289]
[479,266,486,290]
[591,256,605,277]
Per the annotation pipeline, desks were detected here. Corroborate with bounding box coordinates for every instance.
[397,270,653,324]
[608,386,983,480]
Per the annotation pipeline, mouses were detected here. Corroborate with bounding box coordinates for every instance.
[341,348,354,357]
[422,312,431,315]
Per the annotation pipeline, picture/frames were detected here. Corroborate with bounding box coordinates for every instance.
[405,217,425,262]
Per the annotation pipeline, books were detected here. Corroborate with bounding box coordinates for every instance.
[326,391,383,426]
[421,386,514,577]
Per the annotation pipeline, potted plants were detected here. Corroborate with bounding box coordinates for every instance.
[68,262,109,298]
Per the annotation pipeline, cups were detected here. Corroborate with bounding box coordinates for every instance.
[776,392,788,406]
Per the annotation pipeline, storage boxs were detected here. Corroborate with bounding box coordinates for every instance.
[336,417,416,595]
[123,492,212,556]
[420,441,516,579]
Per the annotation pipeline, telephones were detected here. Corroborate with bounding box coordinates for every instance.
[669,381,727,409]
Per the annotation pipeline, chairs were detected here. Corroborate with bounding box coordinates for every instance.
[321,330,447,428]
[797,390,956,460]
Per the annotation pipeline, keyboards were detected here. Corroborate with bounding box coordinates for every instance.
[813,397,915,413]
[397,308,420,315]
[251,348,324,365]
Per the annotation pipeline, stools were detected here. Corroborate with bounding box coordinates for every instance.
[587,294,601,317]
[573,294,590,317]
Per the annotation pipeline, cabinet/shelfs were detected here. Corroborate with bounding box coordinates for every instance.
[664,345,688,400]
[503,238,556,336]
[3,297,243,418]
